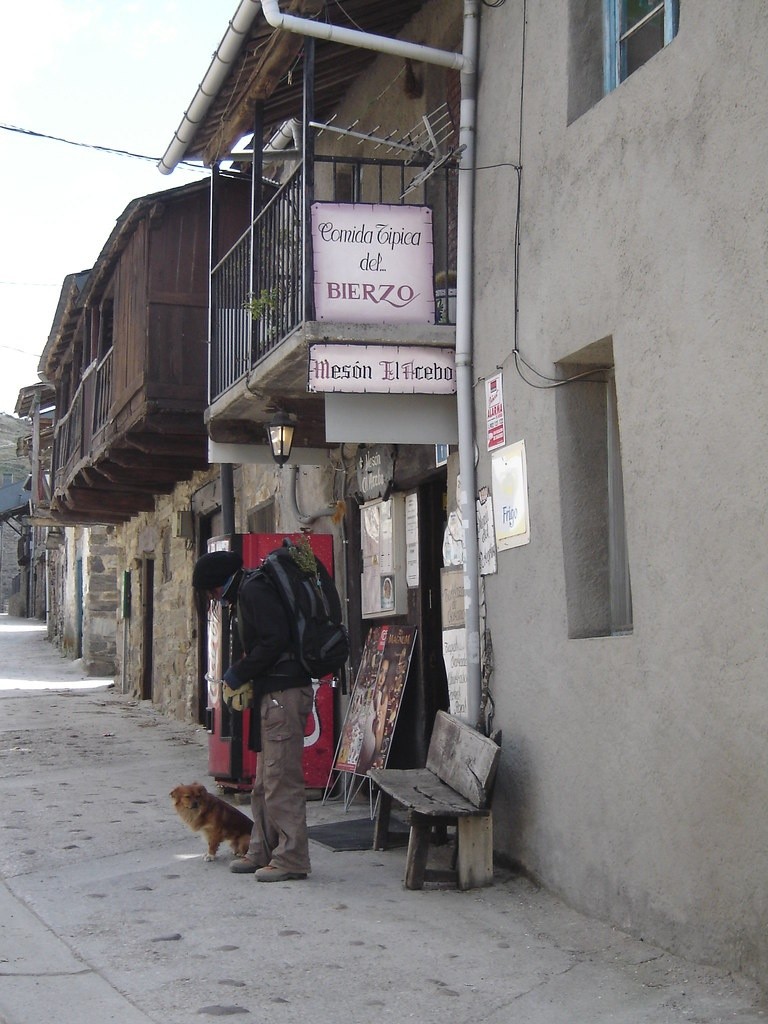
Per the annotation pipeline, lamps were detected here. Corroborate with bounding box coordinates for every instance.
[263,402,299,469]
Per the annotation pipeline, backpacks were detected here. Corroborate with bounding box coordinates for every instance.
[240,537,349,695]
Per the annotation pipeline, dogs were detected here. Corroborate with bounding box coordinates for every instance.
[169,784,254,862]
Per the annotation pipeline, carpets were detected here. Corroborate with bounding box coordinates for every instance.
[306,815,456,855]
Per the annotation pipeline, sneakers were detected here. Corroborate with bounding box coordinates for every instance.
[229,857,263,872]
[255,866,307,881]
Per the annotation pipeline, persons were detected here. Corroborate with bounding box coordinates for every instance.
[192,551,313,882]
[345,653,392,774]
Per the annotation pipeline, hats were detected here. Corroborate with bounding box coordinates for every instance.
[191,550,243,590]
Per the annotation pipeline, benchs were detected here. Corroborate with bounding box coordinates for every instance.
[368,709,503,890]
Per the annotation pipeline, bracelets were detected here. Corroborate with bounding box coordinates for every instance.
[374,752,380,755]
